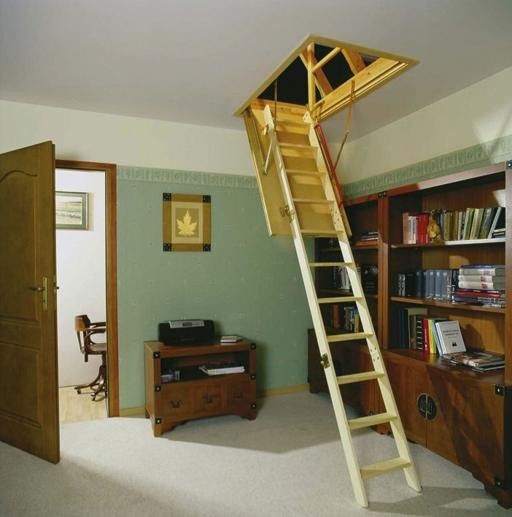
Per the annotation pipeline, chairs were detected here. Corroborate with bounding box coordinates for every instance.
[74,314,109,402]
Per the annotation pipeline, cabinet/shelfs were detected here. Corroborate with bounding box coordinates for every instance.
[142,333,259,438]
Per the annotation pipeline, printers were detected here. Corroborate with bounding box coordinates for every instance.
[158,318,215,347]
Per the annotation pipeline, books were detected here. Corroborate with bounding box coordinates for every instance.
[198,361,246,375]
[396,206,507,376]
[314,228,380,336]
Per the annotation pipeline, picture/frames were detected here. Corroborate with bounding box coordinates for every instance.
[161,192,213,254]
[55,190,90,231]
